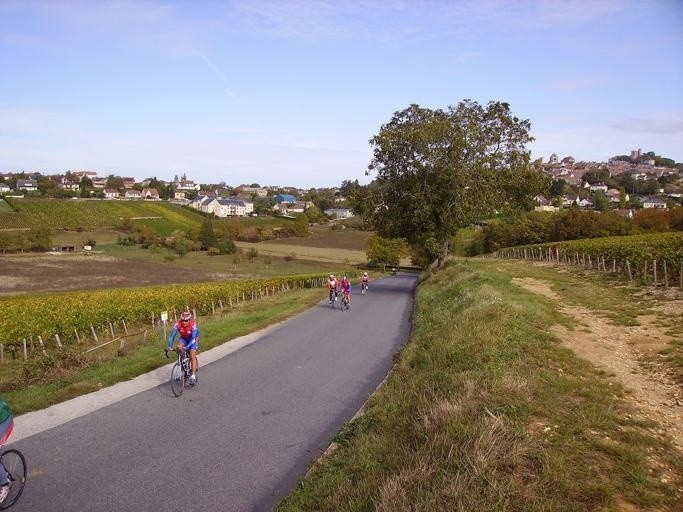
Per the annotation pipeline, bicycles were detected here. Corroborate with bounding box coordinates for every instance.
[328,289,352,312]
[360,282,369,296]
[164,346,200,397]
[0,448,27,511]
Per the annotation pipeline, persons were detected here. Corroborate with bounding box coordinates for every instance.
[168,311,199,385]
[326,274,339,304]
[0,398,14,503]
[360,271,368,294]
[339,276,352,303]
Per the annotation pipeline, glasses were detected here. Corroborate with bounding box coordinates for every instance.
[182,319,189,322]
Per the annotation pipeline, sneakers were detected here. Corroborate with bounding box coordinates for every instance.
[0,479,11,502]
[188,376,197,385]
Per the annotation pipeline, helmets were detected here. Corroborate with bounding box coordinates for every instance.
[180,311,191,320]
[329,275,335,279]
